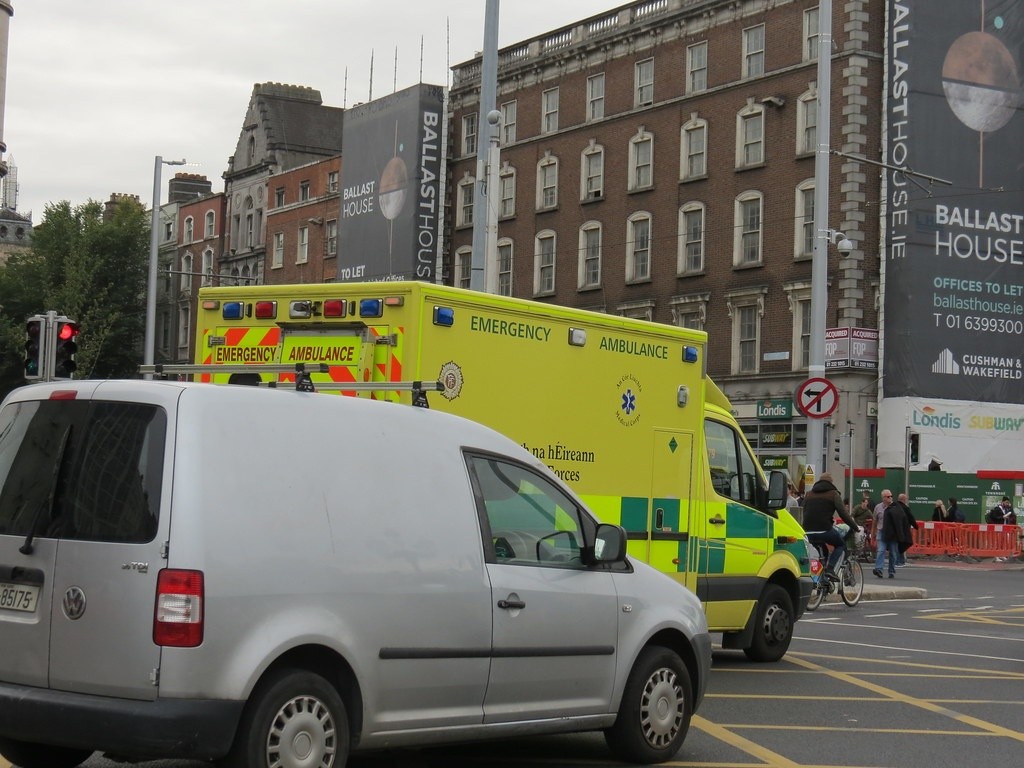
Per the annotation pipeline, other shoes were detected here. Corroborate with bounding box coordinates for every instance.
[889,570,894,577]
[873,569,883,577]
[825,568,840,582]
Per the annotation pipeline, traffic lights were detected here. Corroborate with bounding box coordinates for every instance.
[911,432,919,463]
[49,316,77,383]
[24,317,47,379]
[834,433,850,468]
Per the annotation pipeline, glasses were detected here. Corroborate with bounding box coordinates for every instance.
[884,495,892,498]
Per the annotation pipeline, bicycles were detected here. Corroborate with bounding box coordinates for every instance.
[805,530,864,612]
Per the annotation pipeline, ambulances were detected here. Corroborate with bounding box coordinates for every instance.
[192,279,814,664]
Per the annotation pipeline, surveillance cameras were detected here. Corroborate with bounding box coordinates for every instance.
[837,240,853,257]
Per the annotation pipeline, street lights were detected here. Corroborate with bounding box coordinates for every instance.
[142,155,189,379]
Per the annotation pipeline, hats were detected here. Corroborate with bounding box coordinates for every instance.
[1002,497,1009,501]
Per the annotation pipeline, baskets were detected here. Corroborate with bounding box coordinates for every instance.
[843,532,866,551]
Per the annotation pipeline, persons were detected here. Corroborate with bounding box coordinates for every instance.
[783,481,851,520]
[991,497,1016,562]
[862,490,876,513]
[932,500,950,522]
[851,498,873,533]
[945,497,965,523]
[895,494,908,567]
[802,473,861,596]
[870,489,897,578]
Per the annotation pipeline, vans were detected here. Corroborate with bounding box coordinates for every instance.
[0,374,712,768]
